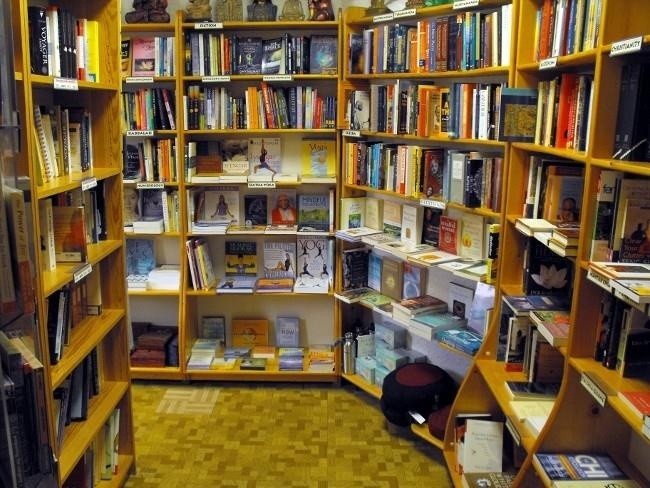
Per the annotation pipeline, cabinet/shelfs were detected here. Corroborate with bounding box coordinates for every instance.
[0,0,58,488]
[339,1,517,450]
[443,0,650,488]
[25,1,136,488]
[179,7,342,387]
[121,11,182,385]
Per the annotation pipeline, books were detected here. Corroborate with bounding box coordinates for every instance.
[455,379,649,488]
[1,5,120,488]
[339,0,512,425]
[185,31,339,374]
[121,32,182,368]
[496,1,650,382]
